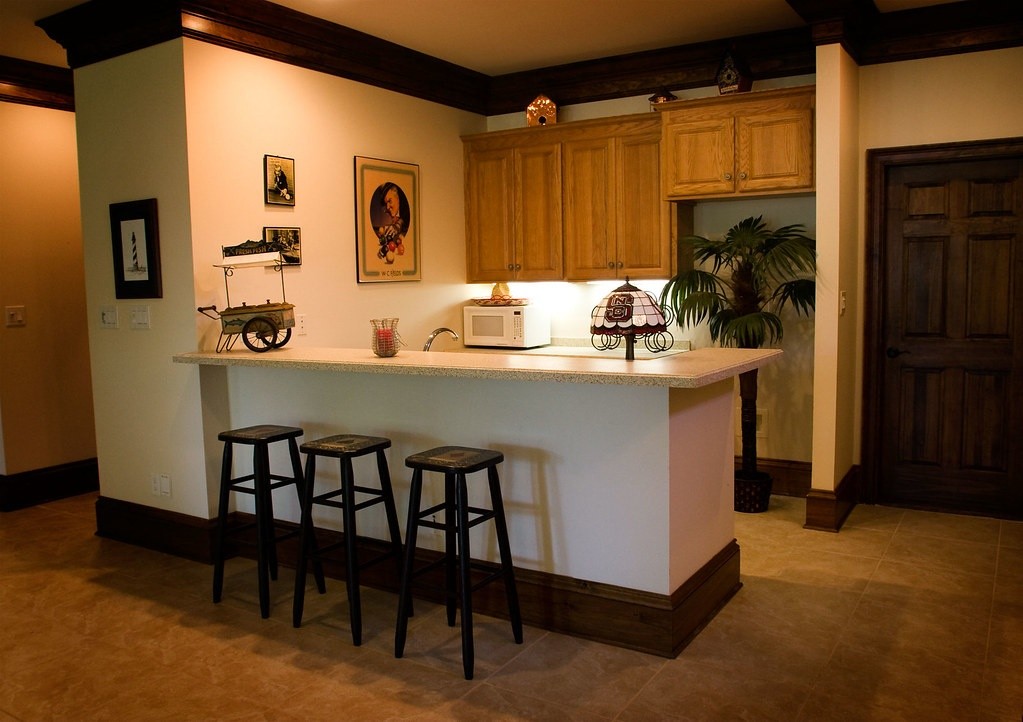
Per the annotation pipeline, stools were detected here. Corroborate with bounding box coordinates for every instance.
[293,435,413,647]
[395,447,522,679]
[212,424,327,618]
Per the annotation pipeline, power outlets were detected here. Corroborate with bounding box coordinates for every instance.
[5,306,26,328]
[151,472,173,500]
[839,292,846,317]
[98,304,119,329]
[128,306,150,329]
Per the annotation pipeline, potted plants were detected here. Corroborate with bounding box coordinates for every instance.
[660,214,816,514]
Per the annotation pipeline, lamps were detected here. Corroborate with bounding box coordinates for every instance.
[590,276,674,361]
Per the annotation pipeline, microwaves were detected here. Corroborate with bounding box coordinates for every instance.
[464,305,552,349]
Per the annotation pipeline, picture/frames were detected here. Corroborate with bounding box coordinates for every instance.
[354,155,422,282]
[264,155,295,207]
[108,198,162,298]
[264,227,302,265]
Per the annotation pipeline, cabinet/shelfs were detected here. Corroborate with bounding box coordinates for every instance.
[651,84,816,197]
[561,112,694,281]
[460,123,562,282]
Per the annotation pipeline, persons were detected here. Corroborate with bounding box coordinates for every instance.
[273,164,288,197]
[269,231,296,252]
[380,182,403,238]
